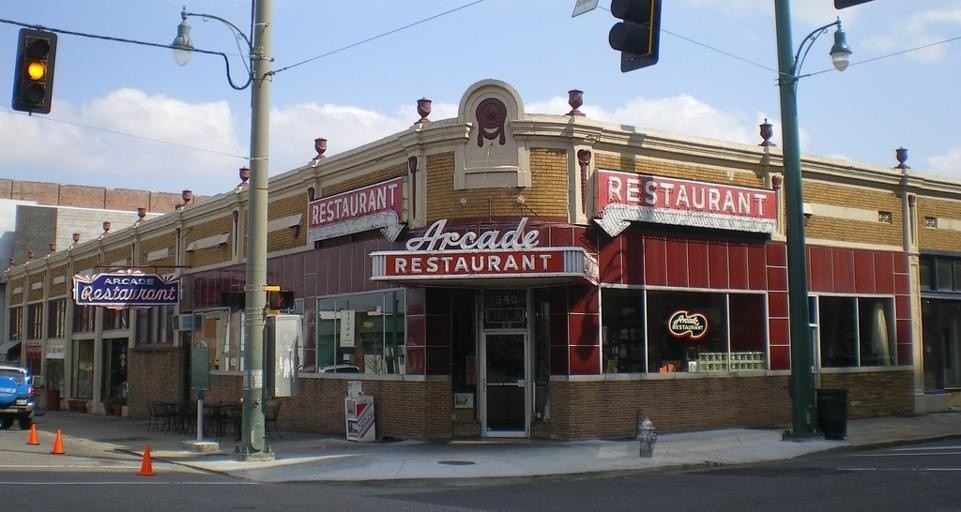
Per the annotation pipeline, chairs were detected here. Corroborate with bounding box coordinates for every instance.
[145,396,286,441]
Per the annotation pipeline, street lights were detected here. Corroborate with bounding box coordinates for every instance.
[174,6,267,458]
[773,14,851,440]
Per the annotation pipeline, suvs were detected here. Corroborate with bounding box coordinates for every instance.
[0,362,34,430]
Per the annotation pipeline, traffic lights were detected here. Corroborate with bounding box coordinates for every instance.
[12,30,56,116]
[609,1,668,70]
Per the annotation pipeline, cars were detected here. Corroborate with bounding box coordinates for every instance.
[318,365,359,374]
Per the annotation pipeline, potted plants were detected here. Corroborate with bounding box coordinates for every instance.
[102,395,127,416]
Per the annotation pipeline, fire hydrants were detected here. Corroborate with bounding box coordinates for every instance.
[638,416,658,460]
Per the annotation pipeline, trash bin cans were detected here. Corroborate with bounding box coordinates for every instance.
[47,390,60,410]
[815,389,849,439]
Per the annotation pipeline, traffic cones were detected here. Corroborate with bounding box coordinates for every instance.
[50,430,71,458]
[25,424,41,446]
[132,441,158,476]
